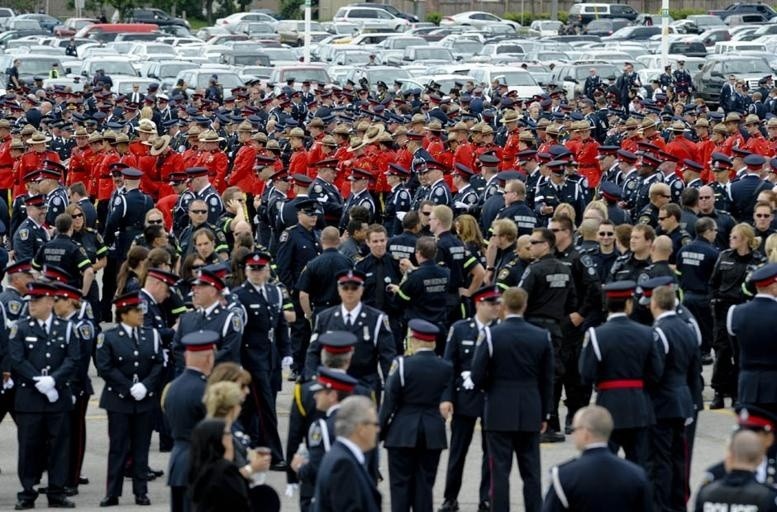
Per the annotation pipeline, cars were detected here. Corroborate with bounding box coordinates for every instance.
[0,1,777,110]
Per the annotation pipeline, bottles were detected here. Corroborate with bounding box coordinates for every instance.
[297,444,311,464]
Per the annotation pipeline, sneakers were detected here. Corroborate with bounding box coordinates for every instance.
[710,396,726,412]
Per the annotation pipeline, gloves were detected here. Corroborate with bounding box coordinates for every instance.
[461,370,477,392]
[129,381,149,401]
[284,482,299,498]
[31,374,60,404]
[2,376,16,391]
[251,470,269,488]
[282,355,294,367]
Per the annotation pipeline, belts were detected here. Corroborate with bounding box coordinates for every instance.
[681,289,707,297]
[597,380,645,391]
[526,317,557,326]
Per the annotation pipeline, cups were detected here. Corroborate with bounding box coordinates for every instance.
[255,445,272,462]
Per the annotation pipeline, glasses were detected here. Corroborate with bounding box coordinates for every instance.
[551,227,566,234]
[147,218,163,224]
[420,211,430,216]
[657,215,672,221]
[70,212,83,218]
[756,213,773,219]
[529,240,548,245]
[598,231,615,236]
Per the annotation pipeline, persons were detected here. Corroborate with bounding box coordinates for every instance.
[98,13,107,23]
[701,431,777,512]
[38,284,94,497]
[316,396,383,512]
[95,293,165,507]
[441,285,502,512]
[292,368,358,512]
[380,320,451,512]
[471,287,555,512]
[66,38,78,55]
[162,331,218,511]
[9,282,81,510]
[544,406,655,512]
[187,363,281,512]
[2,60,775,498]
[695,405,777,512]
[558,15,585,36]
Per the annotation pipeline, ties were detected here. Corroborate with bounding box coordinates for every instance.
[345,313,353,329]
[41,322,48,337]
[131,327,139,351]
[373,260,385,310]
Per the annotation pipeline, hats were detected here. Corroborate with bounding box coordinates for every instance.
[472,284,503,305]
[335,270,367,288]
[3,257,83,301]
[407,318,441,343]
[308,365,359,395]
[733,404,775,434]
[316,330,358,352]
[637,275,677,306]
[180,330,220,353]
[599,279,639,301]
[112,252,272,315]
[747,261,777,288]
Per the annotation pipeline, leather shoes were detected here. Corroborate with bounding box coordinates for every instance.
[47,497,76,508]
[477,501,490,512]
[125,469,164,482]
[135,495,152,506]
[38,475,90,496]
[14,498,34,509]
[437,499,459,511]
[270,460,288,473]
[100,494,119,508]
[538,425,573,444]
